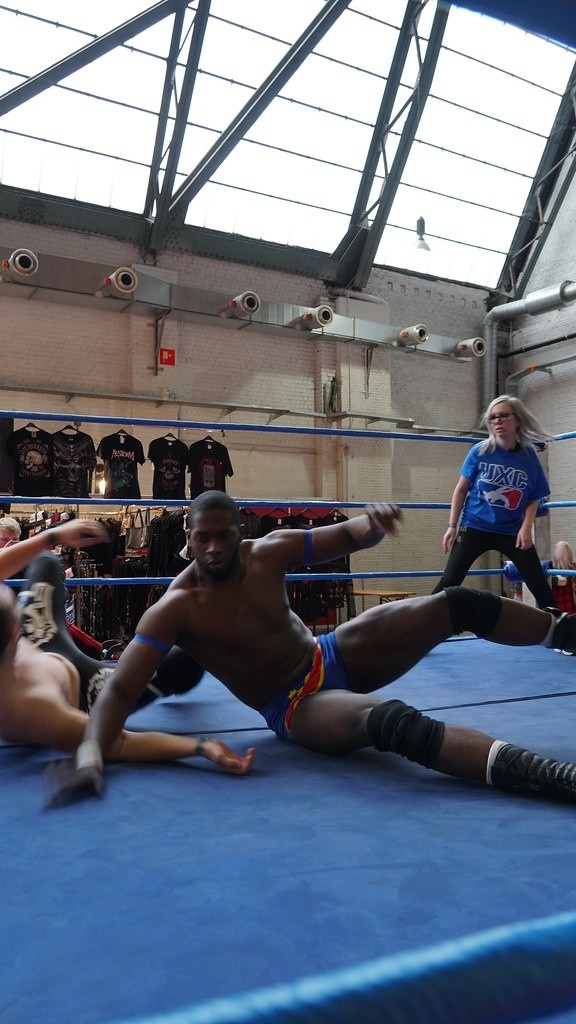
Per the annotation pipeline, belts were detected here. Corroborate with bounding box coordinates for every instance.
[77,563,97,641]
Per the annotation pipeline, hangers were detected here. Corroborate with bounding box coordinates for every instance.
[23,420,40,431]
[266,507,293,518]
[60,422,80,434]
[302,507,320,519]
[116,425,128,436]
[164,428,177,440]
[329,507,346,517]
[242,505,254,515]
[203,433,215,444]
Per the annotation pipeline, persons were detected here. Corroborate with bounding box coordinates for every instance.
[0,517,25,579]
[49,490,576,810]
[0,517,256,774]
[430,395,576,656]
[552,541,576,614]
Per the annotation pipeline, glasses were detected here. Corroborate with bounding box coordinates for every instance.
[487,411,515,424]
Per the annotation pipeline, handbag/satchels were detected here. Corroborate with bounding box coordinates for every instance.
[117,505,154,549]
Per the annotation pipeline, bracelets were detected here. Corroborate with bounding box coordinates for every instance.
[44,530,57,546]
[196,738,205,755]
[448,523,456,527]
[76,741,103,772]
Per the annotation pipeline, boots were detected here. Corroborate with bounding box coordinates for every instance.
[545,606,576,653]
[491,744,576,804]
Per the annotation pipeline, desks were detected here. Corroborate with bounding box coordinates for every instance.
[311,587,417,635]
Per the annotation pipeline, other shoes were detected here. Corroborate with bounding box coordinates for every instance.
[553,648,573,656]
